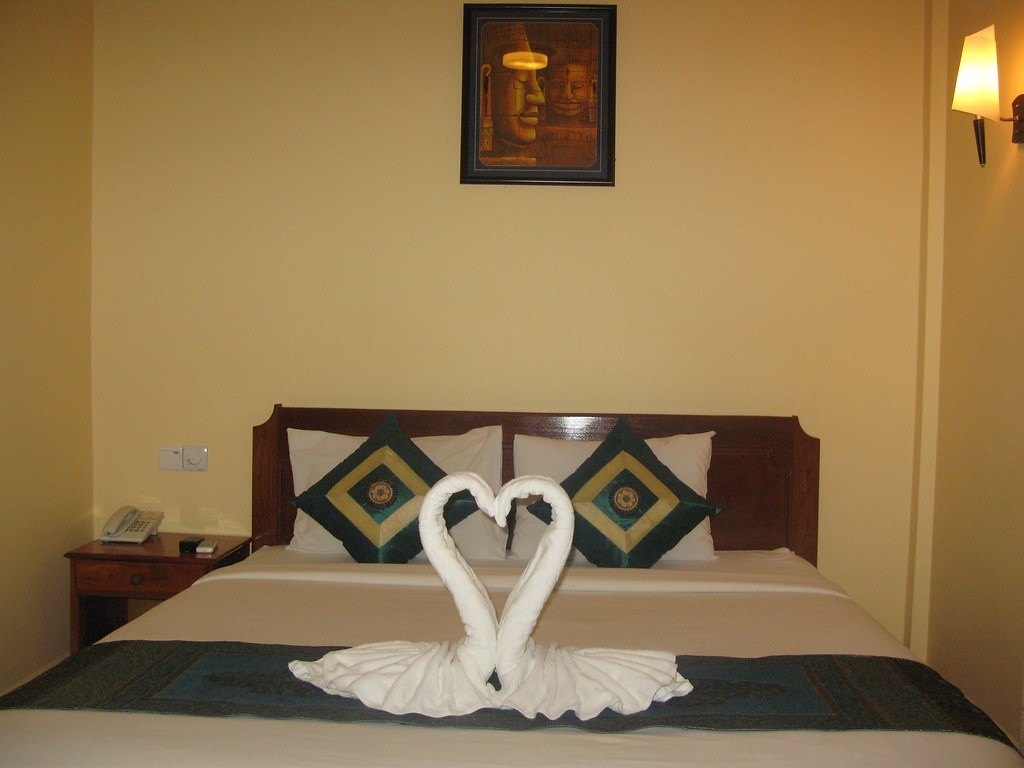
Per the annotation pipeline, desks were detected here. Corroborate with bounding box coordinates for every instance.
[64,531,252,651]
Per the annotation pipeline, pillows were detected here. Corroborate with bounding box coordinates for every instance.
[287,410,480,563]
[287,424,508,561]
[527,416,723,568]
[511,431,719,562]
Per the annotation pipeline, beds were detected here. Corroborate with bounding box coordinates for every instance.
[0,405,1022,768]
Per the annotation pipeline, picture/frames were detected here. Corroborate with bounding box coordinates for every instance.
[461,3,617,187]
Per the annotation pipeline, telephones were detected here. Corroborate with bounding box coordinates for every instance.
[100,505,163,544]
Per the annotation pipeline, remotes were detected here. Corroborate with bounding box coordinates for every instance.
[196,540,217,553]
[179,535,205,553]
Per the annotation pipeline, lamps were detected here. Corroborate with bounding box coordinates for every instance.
[950,23,1024,167]
